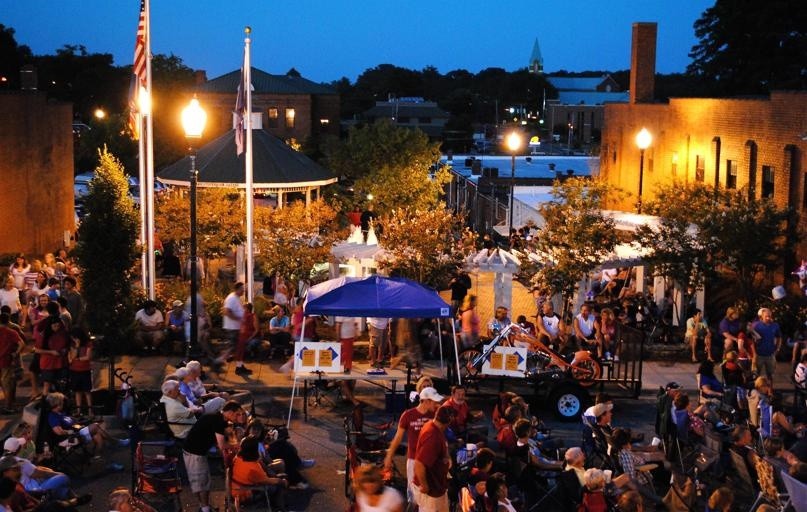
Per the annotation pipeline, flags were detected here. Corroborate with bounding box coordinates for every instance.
[232,47,254,157]
[128,0,147,141]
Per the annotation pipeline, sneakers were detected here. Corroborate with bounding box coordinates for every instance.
[235,365,252,375]
[106,463,124,472]
[78,493,92,505]
[298,459,315,468]
[117,439,130,447]
[290,482,309,490]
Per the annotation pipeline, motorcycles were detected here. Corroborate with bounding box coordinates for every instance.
[452,323,604,390]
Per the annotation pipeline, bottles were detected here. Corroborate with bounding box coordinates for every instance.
[694,478,702,497]
[42,441,50,457]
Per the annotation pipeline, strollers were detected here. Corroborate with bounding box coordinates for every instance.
[135,440,182,510]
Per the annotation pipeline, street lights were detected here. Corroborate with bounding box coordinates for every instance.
[504,129,523,244]
[135,86,153,300]
[178,93,206,371]
[634,128,653,216]
[94,105,106,151]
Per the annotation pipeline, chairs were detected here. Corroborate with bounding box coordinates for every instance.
[136,440,182,501]
[1,403,106,483]
[224,466,277,512]
[340,409,806,512]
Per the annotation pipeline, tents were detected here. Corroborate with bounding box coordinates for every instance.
[286,274,461,430]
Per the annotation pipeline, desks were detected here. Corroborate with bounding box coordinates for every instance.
[295,371,410,425]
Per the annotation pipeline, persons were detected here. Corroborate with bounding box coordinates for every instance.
[1,228,316,511]
[447,259,807,403]
[354,377,807,512]
[366,316,391,366]
[333,317,361,375]
[483,219,544,257]
[338,203,378,241]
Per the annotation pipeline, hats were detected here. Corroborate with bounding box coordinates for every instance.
[593,403,613,417]
[0,456,25,471]
[666,382,683,391]
[4,437,27,454]
[419,387,444,402]
[173,300,184,307]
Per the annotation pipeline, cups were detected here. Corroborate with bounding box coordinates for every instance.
[652,437,661,447]
[603,469,614,484]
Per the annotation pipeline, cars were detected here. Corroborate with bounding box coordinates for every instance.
[71,121,91,139]
[73,171,166,224]
[468,133,589,157]
